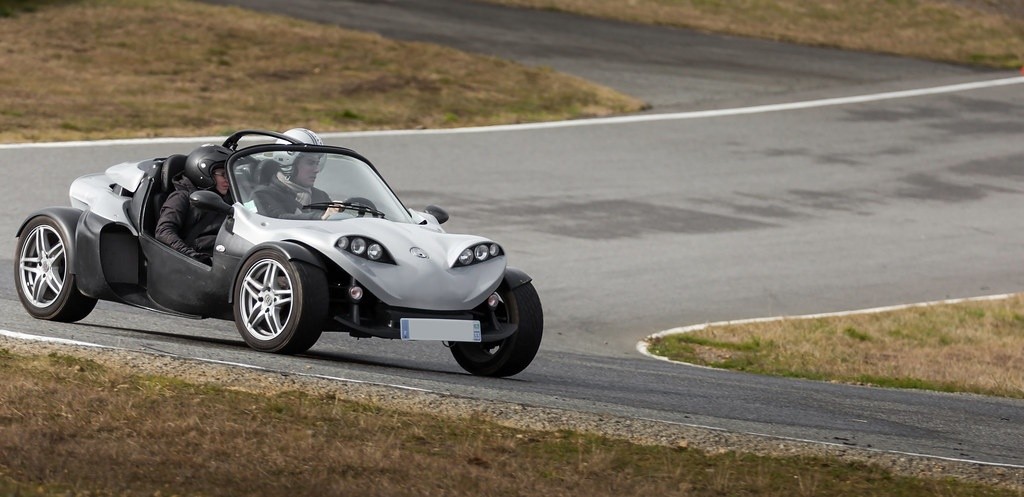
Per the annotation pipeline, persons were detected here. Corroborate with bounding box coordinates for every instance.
[248,128,345,220]
[154,145,235,266]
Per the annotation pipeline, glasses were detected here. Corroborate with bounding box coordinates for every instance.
[213,171,227,179]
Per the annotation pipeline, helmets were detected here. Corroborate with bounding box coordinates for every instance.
[184,144,235,191]
[273,128,327,177]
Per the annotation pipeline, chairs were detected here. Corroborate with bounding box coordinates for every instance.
[153,154,187,223]
[254,158,280,185]
